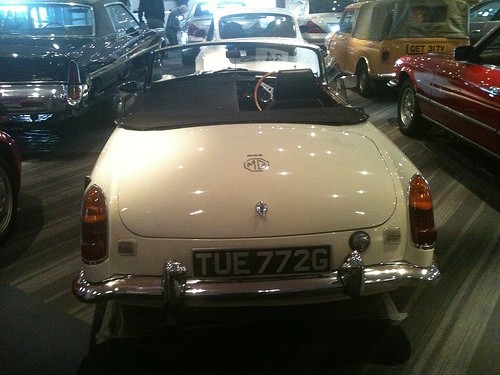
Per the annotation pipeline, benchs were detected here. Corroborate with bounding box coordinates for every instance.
[224,21,292,35]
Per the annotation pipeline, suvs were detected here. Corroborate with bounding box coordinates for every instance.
[324,0,470,96]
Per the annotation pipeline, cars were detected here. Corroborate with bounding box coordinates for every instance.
[293,0,364,60]
[1,0,168,116]
[0,132,22,245]
[181,1,264,65]
[193,6,324,77]
[392,23,500,167]
[471,0,500,44]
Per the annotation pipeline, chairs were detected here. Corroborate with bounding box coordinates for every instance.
[269,69,324,110]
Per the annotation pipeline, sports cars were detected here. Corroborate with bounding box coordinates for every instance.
[70,40,441,311]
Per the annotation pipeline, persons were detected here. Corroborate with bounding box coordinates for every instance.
[138,0,165,29]
[165,5,187,46]
